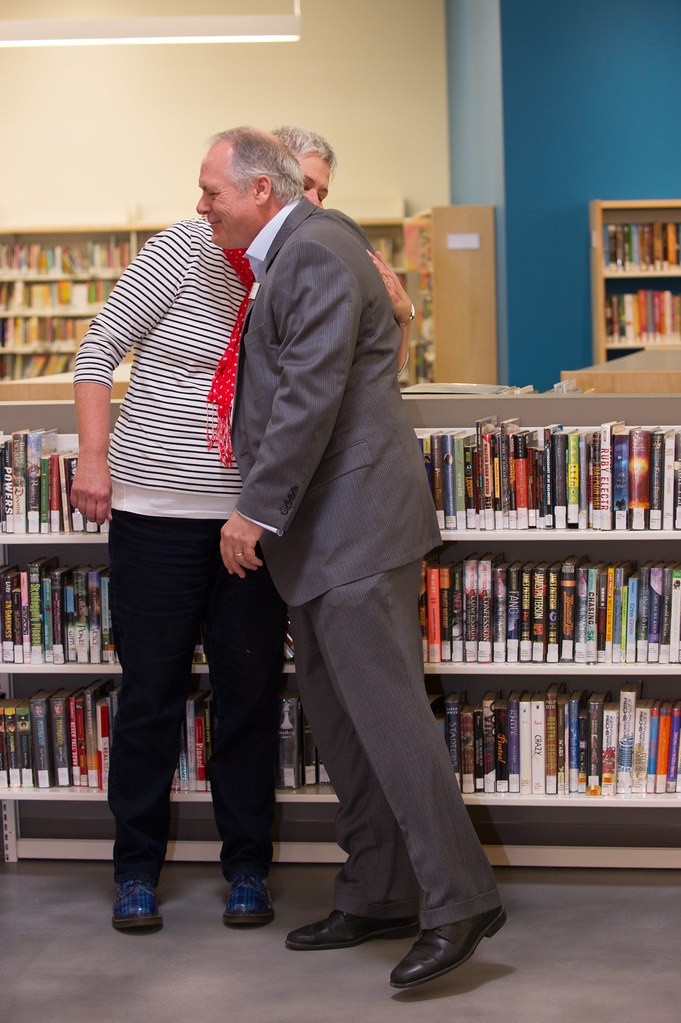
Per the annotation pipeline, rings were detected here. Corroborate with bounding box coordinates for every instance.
[234,553,243,556]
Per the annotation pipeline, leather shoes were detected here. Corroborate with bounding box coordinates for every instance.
[285,906,422,952]
[222,872,275,924]
[389,903,508,988]
[111,880,164,929]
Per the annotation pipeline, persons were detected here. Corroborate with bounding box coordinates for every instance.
[196,126,507,988]
[69,127,416,935]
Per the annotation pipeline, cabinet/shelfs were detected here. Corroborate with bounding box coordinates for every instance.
[0,199,681,874]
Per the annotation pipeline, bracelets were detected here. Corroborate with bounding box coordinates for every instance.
[398,303,416,327]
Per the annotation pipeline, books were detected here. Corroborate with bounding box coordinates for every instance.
[0,222,681,796]
